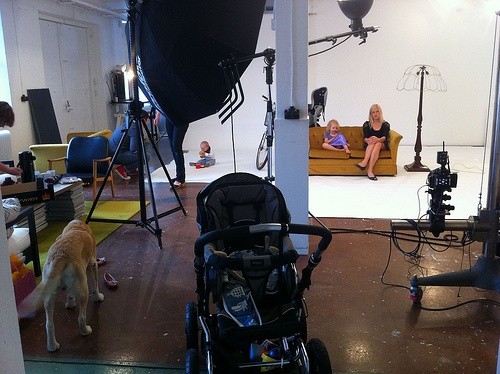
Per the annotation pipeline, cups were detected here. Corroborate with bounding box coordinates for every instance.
[196,163,201,169]
[35,170,56,182]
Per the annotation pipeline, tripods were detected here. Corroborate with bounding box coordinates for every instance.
[85,103,187,250]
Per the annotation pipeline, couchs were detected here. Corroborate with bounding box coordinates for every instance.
[30,130,113,175]
[309,126,403,175]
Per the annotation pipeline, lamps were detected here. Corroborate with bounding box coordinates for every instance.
[398,65,448,172]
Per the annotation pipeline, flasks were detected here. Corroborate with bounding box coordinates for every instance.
[18,151,36,182]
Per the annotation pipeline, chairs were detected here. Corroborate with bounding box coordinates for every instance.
[6,206,41,278]
[47,136,115,205]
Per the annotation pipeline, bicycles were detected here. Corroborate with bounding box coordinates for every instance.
[256,87,328,170]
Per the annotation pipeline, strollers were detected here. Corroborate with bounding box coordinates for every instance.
[184,173,332,374]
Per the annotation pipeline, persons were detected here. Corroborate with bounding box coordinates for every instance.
[0,101,24,177]
[108,110,149,179]
[154,109,189,190]
[354,104,390,180]
[322,119,351,154]
[189,141,216,167]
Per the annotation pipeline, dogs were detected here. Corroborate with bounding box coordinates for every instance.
[37,220,105,352]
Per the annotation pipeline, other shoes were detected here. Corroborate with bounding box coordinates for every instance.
[96,257,107,265]
[355,162,366,170]
[170,184,180,189]
[368,175,377,180]
[103,272,118,288]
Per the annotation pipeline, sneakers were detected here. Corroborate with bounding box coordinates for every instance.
[115,166,131,179]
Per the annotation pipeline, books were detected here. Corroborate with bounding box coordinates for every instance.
[14,184,85,234]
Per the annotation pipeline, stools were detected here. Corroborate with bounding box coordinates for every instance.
[115,113,149,141]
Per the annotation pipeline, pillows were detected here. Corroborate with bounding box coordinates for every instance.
[3,198,21,239]
